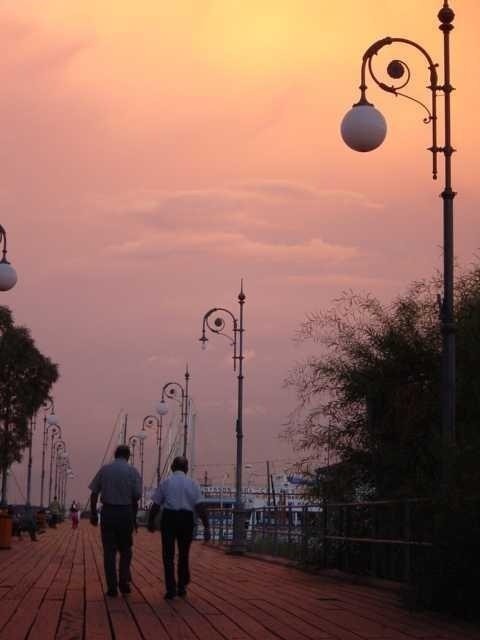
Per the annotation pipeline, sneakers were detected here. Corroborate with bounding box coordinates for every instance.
[104,586,188,600]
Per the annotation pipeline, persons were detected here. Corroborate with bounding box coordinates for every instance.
[69,499,80,530]
[148,456,212,599]
[7,504,46,542]
[48,496,62,528]
[87,444,143,597]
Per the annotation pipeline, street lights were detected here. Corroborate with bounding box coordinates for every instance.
[0,223,18,292]
[198,277,247,554]
[26,396,74,508]
[137,414,162,487]
[220,463,252,507]
[341,0,458,489]
[156,360,191,456]
